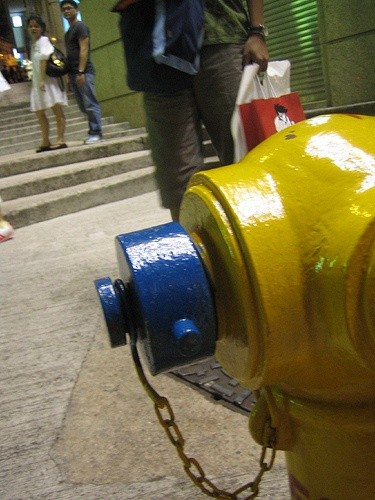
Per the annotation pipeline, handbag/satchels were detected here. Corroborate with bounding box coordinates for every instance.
[46,46,69,78]
[110,0,206,93]
[238,73,308,152]
[230,59,291,163]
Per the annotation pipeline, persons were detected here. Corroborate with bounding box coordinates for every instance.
[110,0,269,222]
[27,16,67,152]
[60,0,103,144]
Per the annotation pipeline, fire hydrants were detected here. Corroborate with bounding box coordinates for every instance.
[93,110,373,498]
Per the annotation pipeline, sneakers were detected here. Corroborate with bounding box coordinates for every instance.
[84,135,105,145]
[0,226,16,242]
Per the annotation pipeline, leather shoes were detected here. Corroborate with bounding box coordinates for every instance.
[36,143,68,152]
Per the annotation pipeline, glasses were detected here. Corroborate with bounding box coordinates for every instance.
[61,7,75,12]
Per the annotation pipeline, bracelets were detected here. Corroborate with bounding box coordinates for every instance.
[76,70,84,75]
[248,24,268,38]
